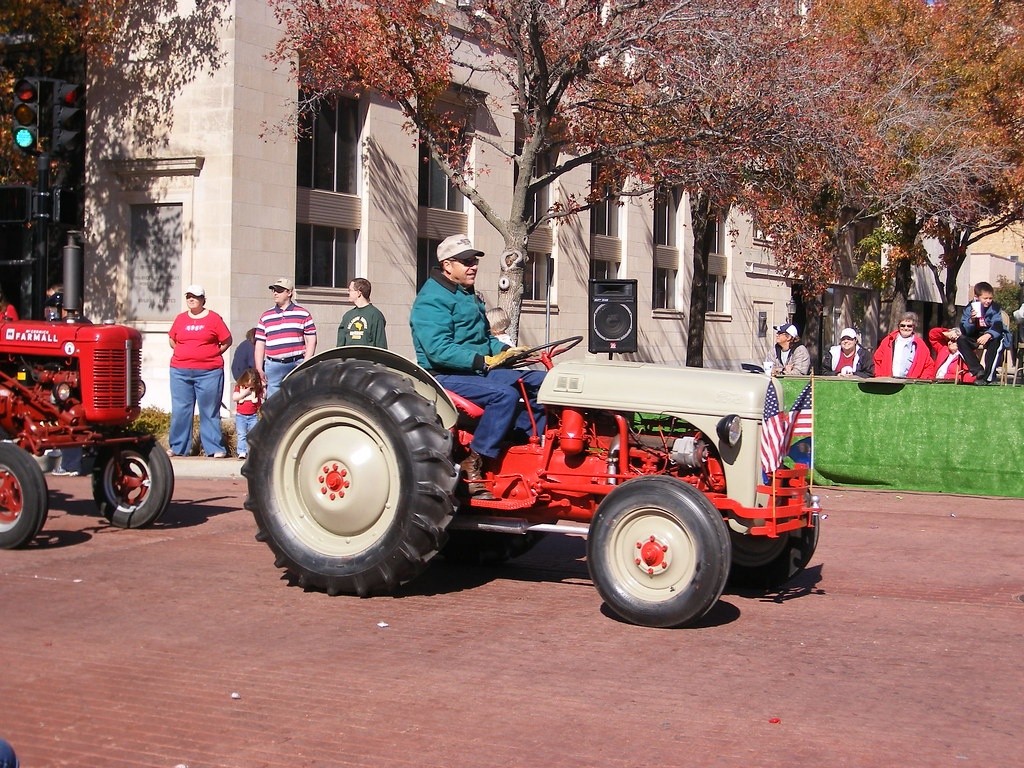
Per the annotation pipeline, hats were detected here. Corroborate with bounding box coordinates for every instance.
[437,234,485,262]
[840,328,857,339]
[184,285,205,297]
[268,278,294,292]
[773,324,798,338]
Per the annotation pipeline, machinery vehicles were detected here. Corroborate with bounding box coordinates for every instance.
[241,336,820,629]
[1,229,175,550]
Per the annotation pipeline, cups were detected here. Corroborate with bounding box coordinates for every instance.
[971,301,982,318]
[762,362,773,376]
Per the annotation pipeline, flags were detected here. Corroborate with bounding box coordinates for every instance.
[761,376,788,485]
[787,380,811,449]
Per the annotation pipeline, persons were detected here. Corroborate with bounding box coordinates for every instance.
[823,327,875,378]
[336,277,388,349]
[231,327,257,382]
[873,312,935,380]
[45,284,93,477]
[957,282,1003,386]
[409,233,548,500]
[254,277,317,400]
[927,327,977,382]
[766,324,810,376]
[233,368,267,458]
[1013,303,1024,325]
[165,284,233,458]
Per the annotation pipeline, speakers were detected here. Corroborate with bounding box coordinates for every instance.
[588,278,638,355]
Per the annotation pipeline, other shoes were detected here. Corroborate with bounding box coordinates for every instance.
[238,452,247,459]
[166,448,178,457]
[214,452,227,458]
[974,370,989,386]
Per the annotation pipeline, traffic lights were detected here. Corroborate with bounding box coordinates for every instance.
[0,186,33,224]
[12,78,47,152]
[51,81,86,158]
[55,187,80,226]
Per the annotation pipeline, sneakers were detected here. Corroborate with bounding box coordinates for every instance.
[459,451,495,500]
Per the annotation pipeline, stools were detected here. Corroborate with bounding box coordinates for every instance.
[444,388,485,419]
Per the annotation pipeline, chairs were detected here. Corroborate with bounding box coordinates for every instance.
[955,311,1024,386]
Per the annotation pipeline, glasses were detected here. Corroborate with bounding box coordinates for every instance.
[448,259,479,267]
[186,295,196,299]
[272,286,285,293]
[900,324,914,328]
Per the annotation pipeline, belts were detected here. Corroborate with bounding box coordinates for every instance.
[266,354,304,364]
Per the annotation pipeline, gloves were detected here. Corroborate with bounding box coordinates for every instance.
[485,345,539,371]
